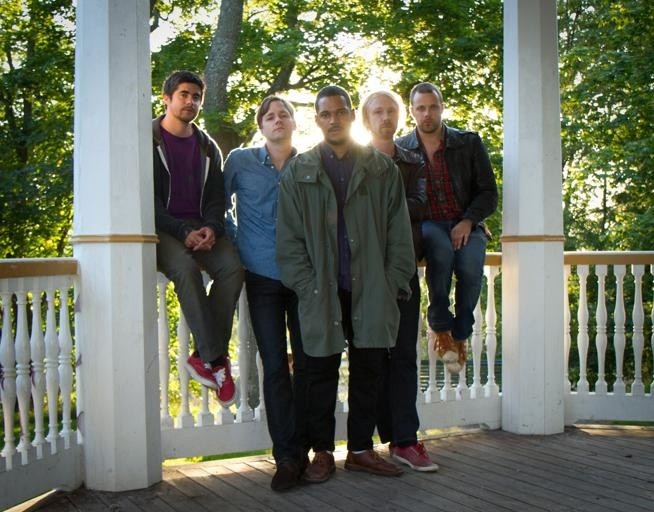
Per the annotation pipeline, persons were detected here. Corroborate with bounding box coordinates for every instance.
[395,82,498,375]
[151,71,244,407]
[221,96,312,494]
[353,90,440,473]
[276,86,416,483]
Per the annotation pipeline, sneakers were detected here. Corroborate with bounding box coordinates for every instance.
[185,353,218,390]
[433,330,466,372]
[390,442,440,472]
[270,452,335,490]
[345,451,403,476]
[212,358,237,408]
